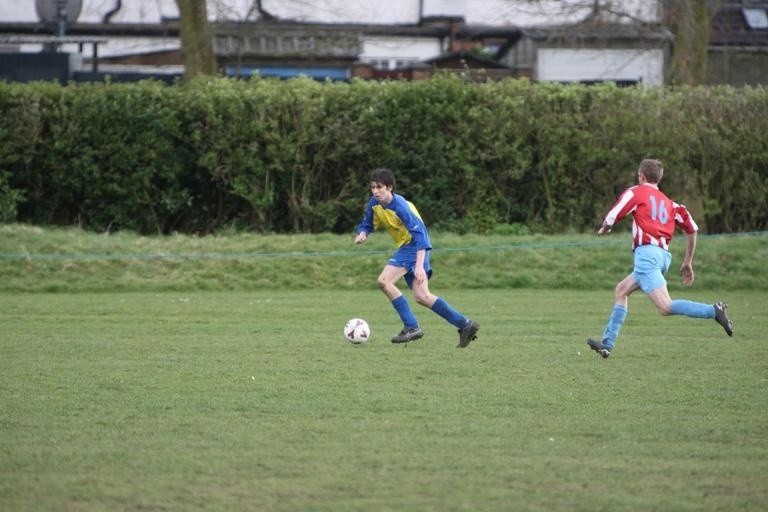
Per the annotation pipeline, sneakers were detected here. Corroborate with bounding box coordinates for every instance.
[391,327,424,343]
[714,301,733,337]
[456,320,479,348]
[587,338,611,358]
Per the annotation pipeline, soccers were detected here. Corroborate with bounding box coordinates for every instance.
[343,318,370,344]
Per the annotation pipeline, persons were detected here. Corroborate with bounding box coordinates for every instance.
[354,168,481,347]
[586,158,733,358]
